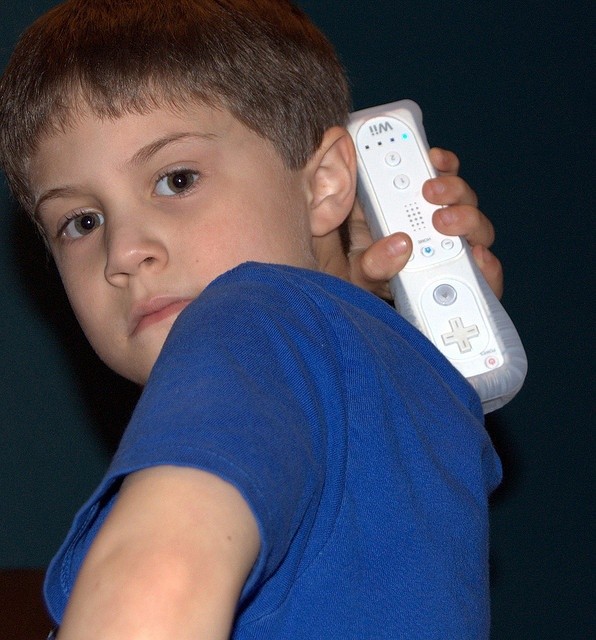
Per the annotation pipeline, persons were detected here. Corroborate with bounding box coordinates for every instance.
[2,1,503,636]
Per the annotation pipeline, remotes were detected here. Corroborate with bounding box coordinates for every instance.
[342,98,529,425]
